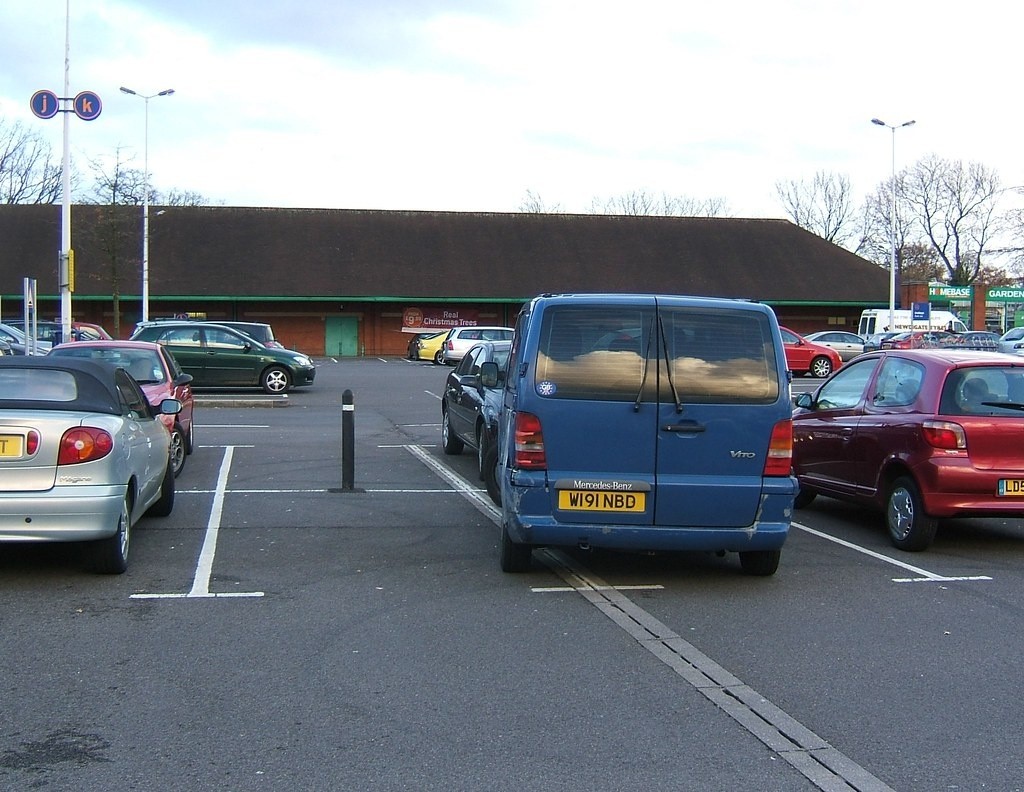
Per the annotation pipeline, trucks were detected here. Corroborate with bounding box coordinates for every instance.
[858,309,968,341]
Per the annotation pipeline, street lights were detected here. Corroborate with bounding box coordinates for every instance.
[871,118,916,331]
[120,86,175,322]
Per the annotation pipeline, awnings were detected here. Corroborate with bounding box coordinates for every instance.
[6,296,901,307]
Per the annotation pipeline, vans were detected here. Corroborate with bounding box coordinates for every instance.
[480,293,800,577]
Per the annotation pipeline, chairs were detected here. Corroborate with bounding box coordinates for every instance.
[963,377,990,404]
[896,378,920,403]
[131,358,154,381]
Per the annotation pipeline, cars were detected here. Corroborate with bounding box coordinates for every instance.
[1,355,183,576]
[128,321,315,394]
[998,327,1024,356]
[792,349,1024,553]
[779,325,843,378]
[407,333,433,361]
[417,331,451,366]
[881,332,964,350]
[863,333,901,353]
[33,340,195,478]
[803,331,868,362]
[442,340,512,481]
[0,320,113,355]
[946,331,1002,350]
[442,326,515,362]
